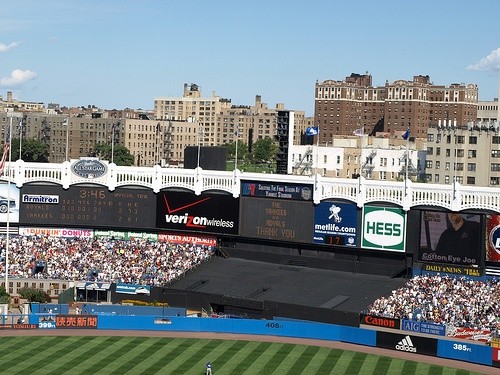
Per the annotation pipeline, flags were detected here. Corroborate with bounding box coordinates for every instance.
[369,116,383,136]
[304,126,320,136]
[352,127,364,135]
[402,129,410,140]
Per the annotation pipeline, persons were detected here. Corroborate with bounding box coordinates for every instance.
[48,308,52,314]
[76,306,79,315]
[0,233,213,288]
[206,362,212,375]
[432,212,479,266]
[364,273,500,346]
[18,317,24,325]
